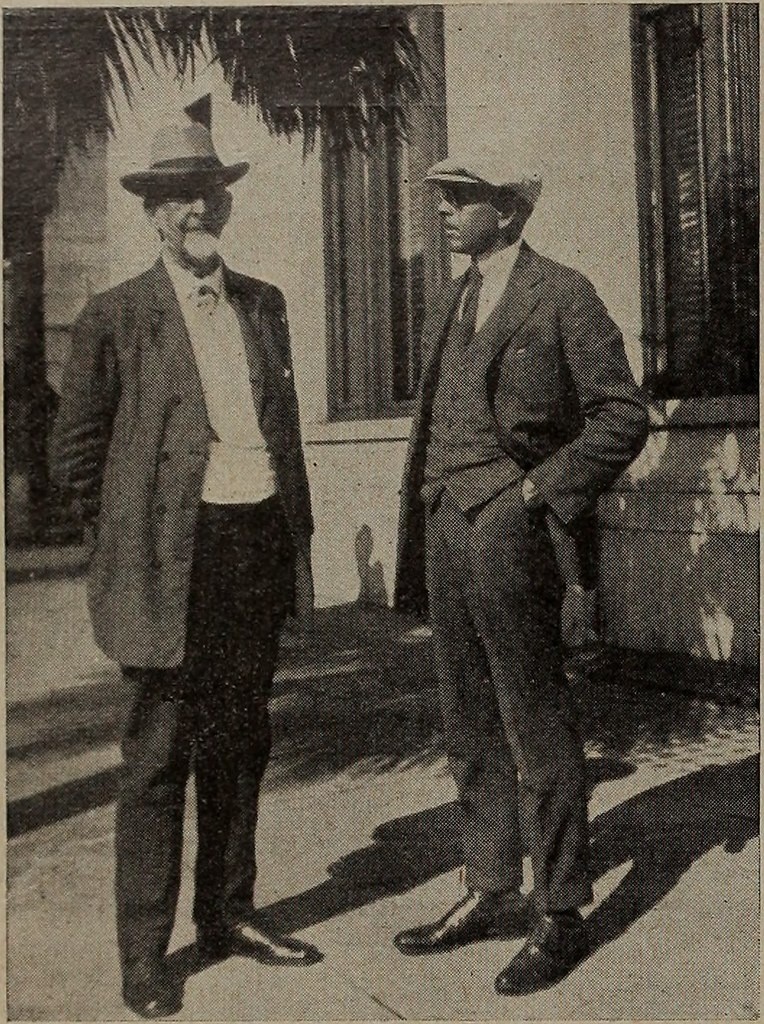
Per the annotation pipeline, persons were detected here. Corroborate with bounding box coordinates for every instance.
[50,123,324,1019]
[393,145,650,997]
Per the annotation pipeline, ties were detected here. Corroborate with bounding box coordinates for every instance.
[460,264,485,344]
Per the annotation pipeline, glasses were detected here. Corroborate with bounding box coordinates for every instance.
[155,181,215,203]
[440,186,485,203]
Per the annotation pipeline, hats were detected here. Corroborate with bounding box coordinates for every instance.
[120,116,252,195]
[425,149,545,205]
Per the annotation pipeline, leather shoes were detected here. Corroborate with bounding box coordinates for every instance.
[496,910,590,996]
[193,917,319,966]
[121,951,181,1015]
[394,889,532,955]
